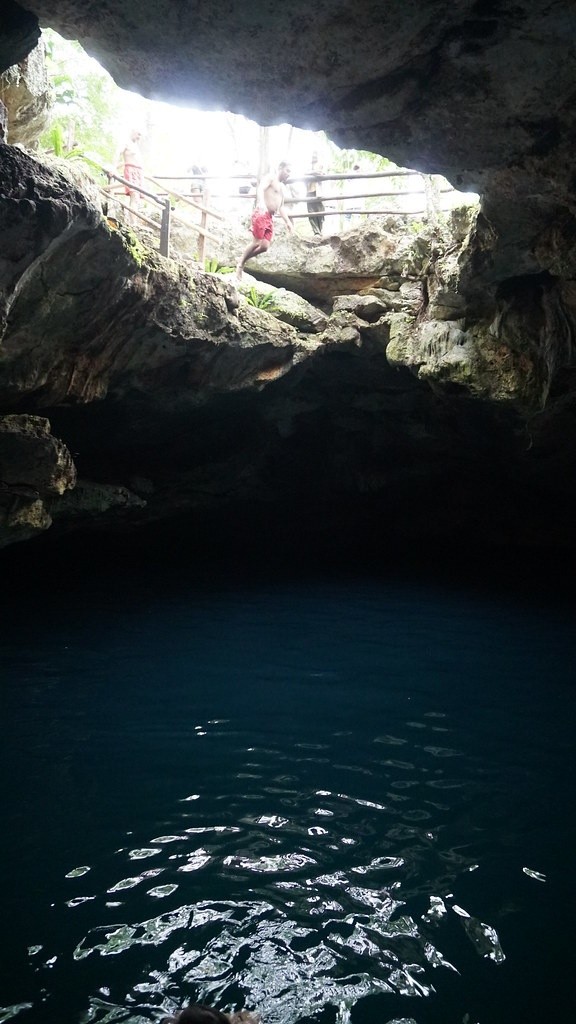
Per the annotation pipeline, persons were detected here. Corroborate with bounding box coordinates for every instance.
[342,165,366,233]
[236,161,294,280]
[304,155,326,234]
[187,155,210,204]
[110,128,144,226]
